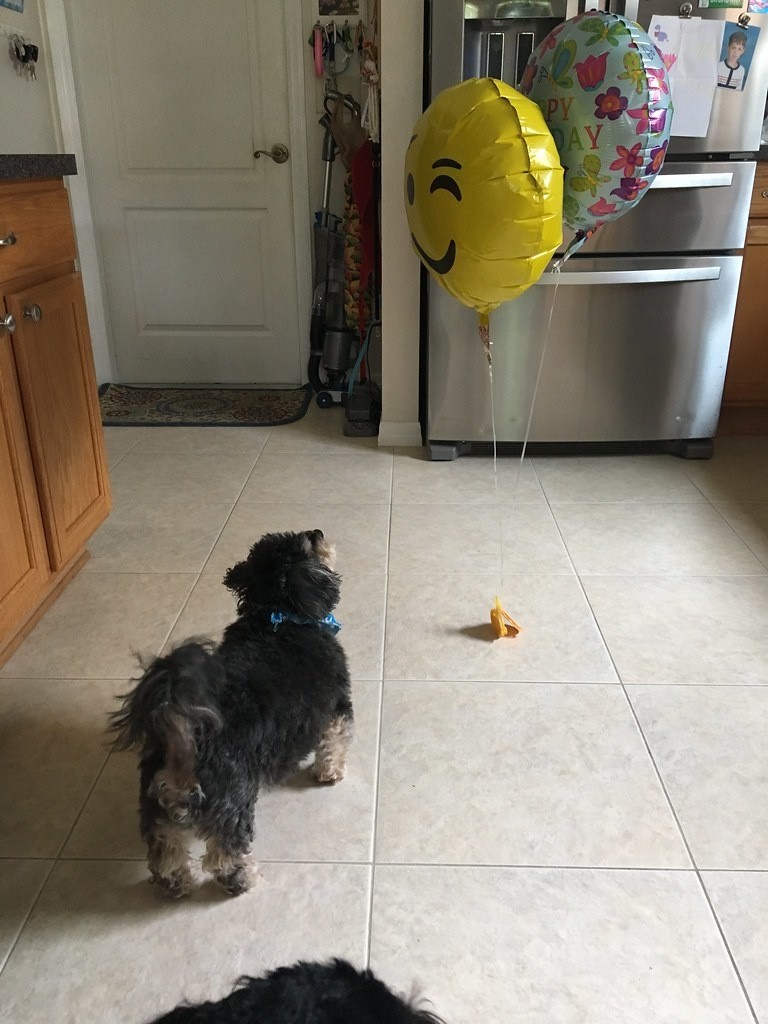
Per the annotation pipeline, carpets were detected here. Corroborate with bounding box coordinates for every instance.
[97,380,313,424]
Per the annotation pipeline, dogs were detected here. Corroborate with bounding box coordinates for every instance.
[103,529,353,897]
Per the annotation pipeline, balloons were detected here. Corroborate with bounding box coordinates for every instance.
[404,73,564,313]
[522,11,672,239]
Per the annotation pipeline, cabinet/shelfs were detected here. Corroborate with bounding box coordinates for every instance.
[0,178,110,663]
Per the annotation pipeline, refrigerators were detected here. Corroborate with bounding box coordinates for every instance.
[427,0,768,459]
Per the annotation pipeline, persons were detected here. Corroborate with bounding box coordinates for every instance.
[717,31,747,92]
